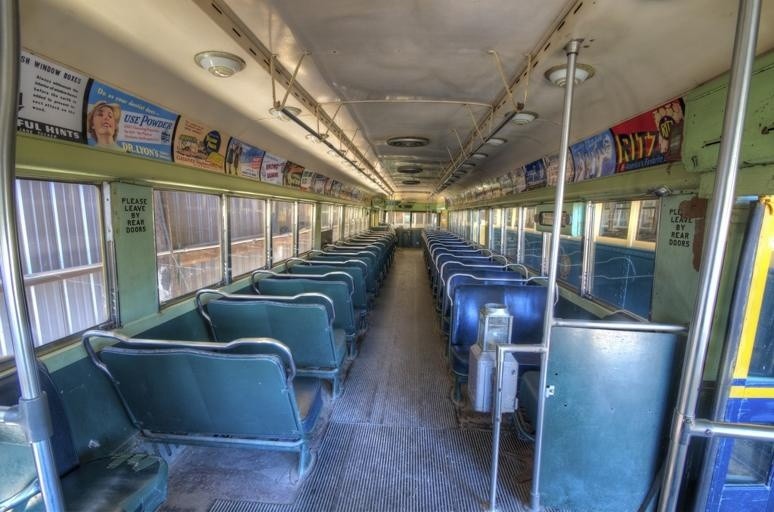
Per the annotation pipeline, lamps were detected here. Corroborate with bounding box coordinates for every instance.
[193,51,394,198]
[432,58,597,205]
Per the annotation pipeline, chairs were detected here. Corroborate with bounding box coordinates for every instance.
[80,330,329,486]
[194,219,399,401]
[417,222,660,485]
[1,359,170,512]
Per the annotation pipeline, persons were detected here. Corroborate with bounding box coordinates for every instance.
[654,100,683,153]
[225,144,235,176]
[234,143,242,175]
[87,101,121,152]
[574,137,616,181]
